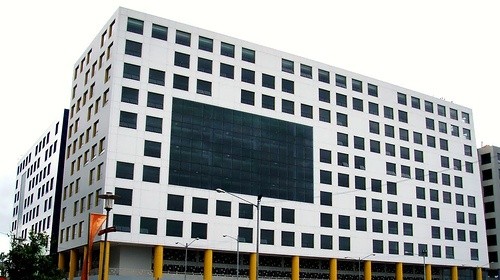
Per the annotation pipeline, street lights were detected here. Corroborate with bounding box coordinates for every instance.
[222,234,241,277]
[175,237,200,280]
[216,187,264,280]
[97,192,121,279]
